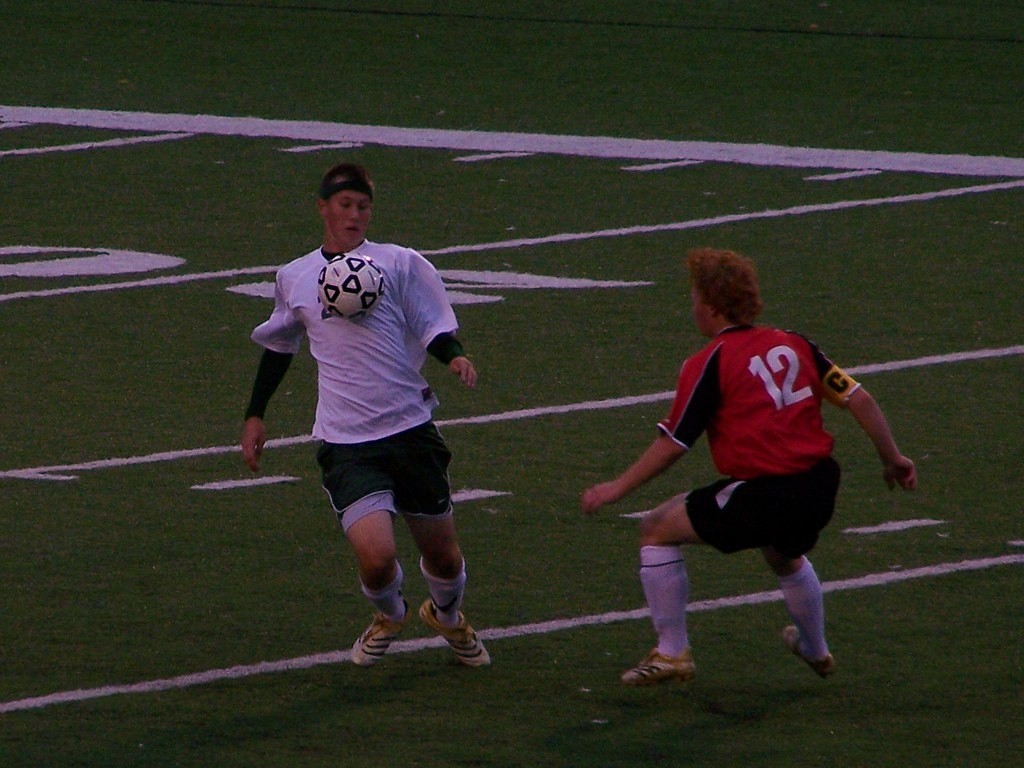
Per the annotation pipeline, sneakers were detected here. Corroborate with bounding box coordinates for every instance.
[351,590,412,667]
[419,599,491,667]
[621,647,697,687]
[782,625,834,676]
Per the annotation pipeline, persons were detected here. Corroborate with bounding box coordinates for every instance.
[240,158,492,668]
[582,248,918,684]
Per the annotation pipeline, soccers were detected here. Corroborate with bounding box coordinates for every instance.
[316,250,386,321]
